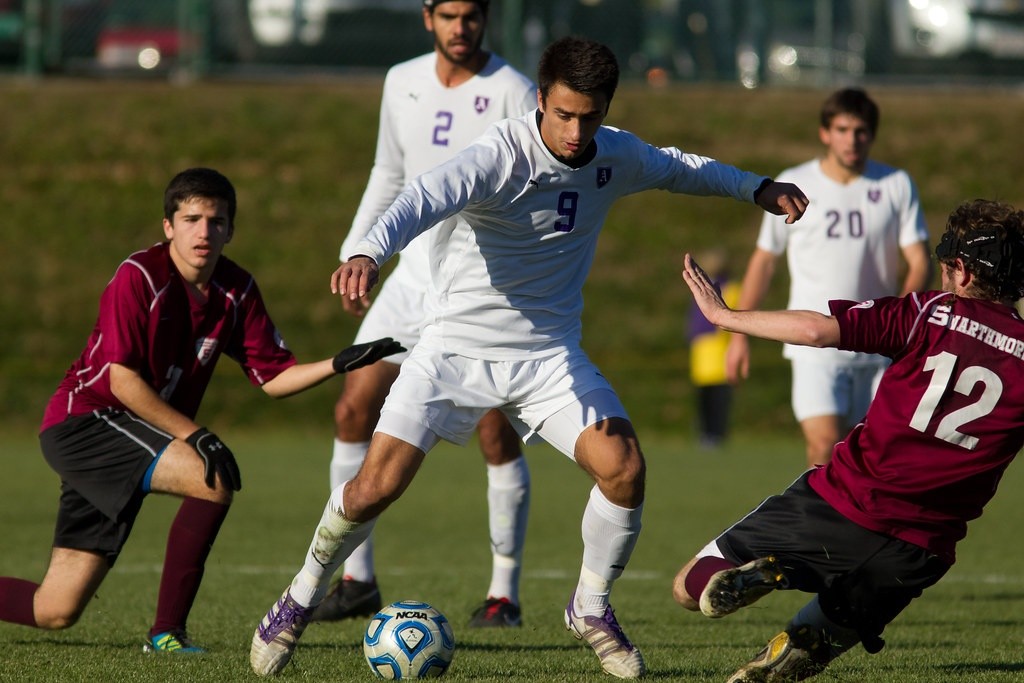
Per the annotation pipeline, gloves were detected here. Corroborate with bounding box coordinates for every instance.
[185,427,242,491]
[333,338,407,374]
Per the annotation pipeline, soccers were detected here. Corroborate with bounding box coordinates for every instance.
[363,599,455,682]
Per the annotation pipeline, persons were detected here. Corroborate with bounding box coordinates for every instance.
[674,197,1024,683]
[0,168,406,651]
[728,86,931,468]
[689,250,748,446]
[304,0,539,627]
[251,37,809,679]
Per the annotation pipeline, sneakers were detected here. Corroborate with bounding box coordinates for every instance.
[311,577,380,621]
[564,590,645,679]
[700,556,785,618]
[726,624,825,683]
[464,597,522,628]
[143,627,207,653]
[249,585,320,676]
